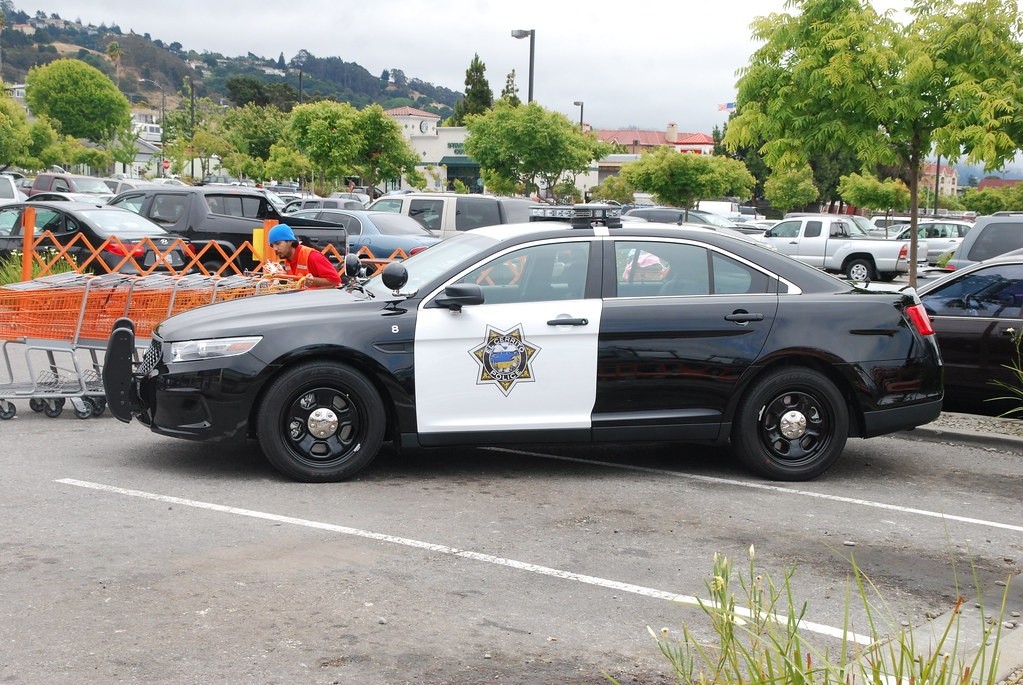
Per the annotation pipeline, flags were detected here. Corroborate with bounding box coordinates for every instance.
[718,103,734,112]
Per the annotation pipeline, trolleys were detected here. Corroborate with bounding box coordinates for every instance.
[0,272,315,421]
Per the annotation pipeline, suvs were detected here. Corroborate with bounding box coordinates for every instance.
[943,211,1023,273]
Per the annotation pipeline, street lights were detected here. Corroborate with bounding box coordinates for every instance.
[138,78,165,179]
[510,28,536,107]
[573,101,584,136]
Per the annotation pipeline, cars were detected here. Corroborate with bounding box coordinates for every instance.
[916,248,1023,417]
[102,202,948,483]
[0,170,973,421]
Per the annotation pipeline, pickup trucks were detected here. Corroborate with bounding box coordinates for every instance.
[747,218,928,282]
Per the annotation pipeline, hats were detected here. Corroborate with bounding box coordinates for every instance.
[268,223,297,247]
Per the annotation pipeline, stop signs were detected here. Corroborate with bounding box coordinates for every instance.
[162,161,170,169]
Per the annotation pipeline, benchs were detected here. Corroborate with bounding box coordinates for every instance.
[659,276,742,294]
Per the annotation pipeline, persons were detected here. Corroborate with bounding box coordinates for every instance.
[267,224,342,288]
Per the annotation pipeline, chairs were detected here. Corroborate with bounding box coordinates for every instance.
[559,262,586,301]
[517,261,553,300]
